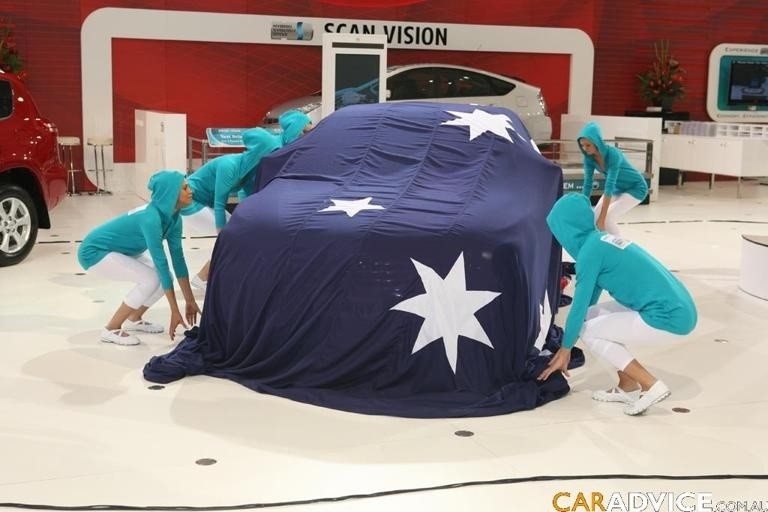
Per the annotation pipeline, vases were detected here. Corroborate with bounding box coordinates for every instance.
[653,96,676,115]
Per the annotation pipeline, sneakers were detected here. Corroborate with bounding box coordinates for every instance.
[590,386,641,404]
[190,273,208,291]
[621,378,671,417]
[98,326,141,346]
[122,319,165,335]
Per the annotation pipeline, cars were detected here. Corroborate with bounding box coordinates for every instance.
[262,63,552,149]
[0,69,70,265]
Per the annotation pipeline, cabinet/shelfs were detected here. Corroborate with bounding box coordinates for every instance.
[660,135,768,199]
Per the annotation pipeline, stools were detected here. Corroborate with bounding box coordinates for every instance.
[57,136,116,197]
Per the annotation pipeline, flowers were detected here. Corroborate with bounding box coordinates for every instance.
[634,37,689,101]
[1,8,24,75]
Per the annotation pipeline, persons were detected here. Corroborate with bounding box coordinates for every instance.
[77,171,201,346]
[181,127,280,291]
[576,123,649,237]
[537,192,698,415]
[237,108,314,204]
